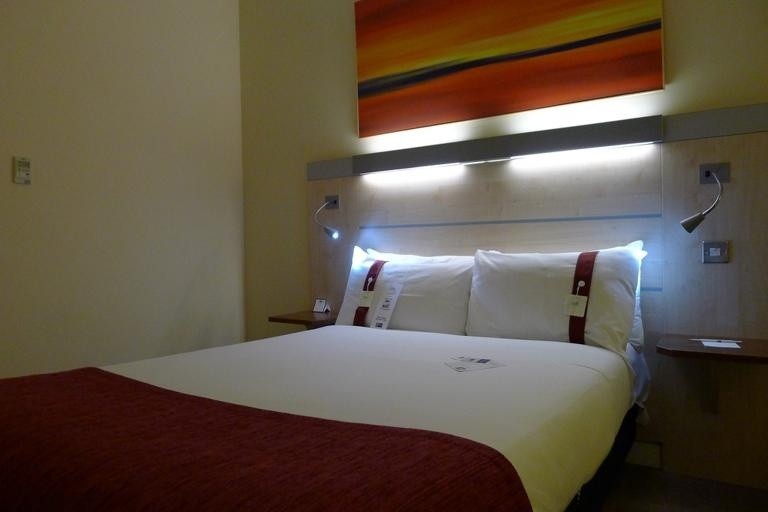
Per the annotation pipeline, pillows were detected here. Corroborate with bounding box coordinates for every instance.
[335,245,476,335]
[465,239,644,362]
[367,248,472,265]
[630,250,648,354]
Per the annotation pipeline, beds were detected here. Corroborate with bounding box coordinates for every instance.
[0,324,652,512]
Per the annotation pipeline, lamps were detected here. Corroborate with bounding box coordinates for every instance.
[679,159,732,234]
[312,194,341,240]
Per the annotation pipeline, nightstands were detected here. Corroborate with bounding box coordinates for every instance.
[655,335,768,414]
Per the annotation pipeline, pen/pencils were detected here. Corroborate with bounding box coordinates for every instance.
[687,338,744,343]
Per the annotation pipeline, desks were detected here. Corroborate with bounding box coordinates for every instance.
[268,310,338,330]
